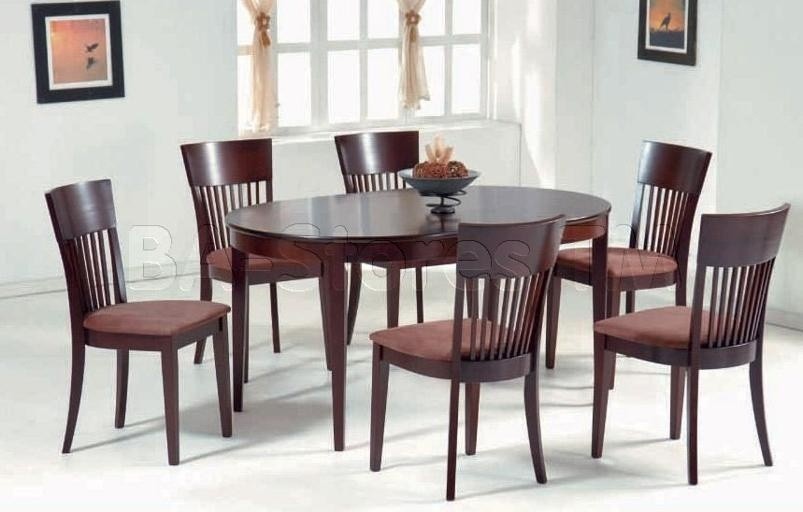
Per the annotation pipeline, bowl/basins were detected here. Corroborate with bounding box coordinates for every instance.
[397,167,482,194]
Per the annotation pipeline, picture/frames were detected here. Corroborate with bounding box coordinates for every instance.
[29,3,128,109]
[636,1,697,67]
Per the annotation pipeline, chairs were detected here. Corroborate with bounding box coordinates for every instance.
[40,120,791,504]
